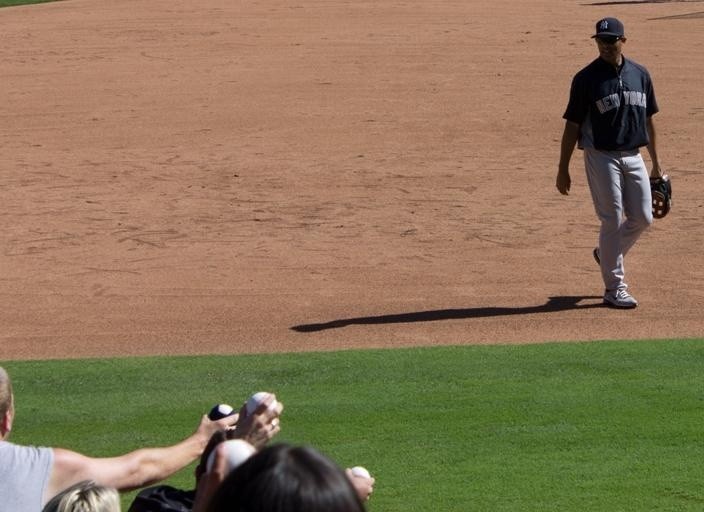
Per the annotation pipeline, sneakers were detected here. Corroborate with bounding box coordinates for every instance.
[603,288,637,307]
[593,247,601,265]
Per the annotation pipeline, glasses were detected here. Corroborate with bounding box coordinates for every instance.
[595,36,622,44]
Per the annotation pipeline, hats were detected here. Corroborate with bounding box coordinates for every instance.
[591,18,624,38]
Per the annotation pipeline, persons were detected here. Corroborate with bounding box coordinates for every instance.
[556,17,672,309]
[0,366,240,512]
[128,390,284,512]
[41,445,224,512]
[208,443,375,512]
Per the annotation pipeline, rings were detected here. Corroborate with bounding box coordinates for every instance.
[270,421,277,427]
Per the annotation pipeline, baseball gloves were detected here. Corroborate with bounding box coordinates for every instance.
[648,168,673,219]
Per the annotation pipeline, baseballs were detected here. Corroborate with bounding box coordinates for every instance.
[246,391,278,418]
[207,440,257,483]
[351,465,371,481]
[209,402,238,421]
